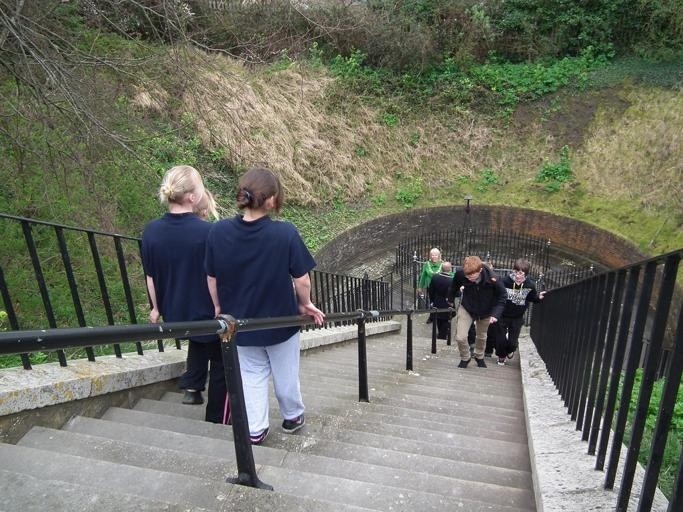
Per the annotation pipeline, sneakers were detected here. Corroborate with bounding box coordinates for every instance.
[181,388,204,405]
[280,414,305,433]
[457,354,518,368]
[250,426,269,446]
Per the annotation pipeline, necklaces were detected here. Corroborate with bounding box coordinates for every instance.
[427,262,444,274]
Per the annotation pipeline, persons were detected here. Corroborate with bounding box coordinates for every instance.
[416,247,454,324]
[138,163,233,424]
[205,167,326,445]
[427,262,455,342]
[446,254,547,369]
